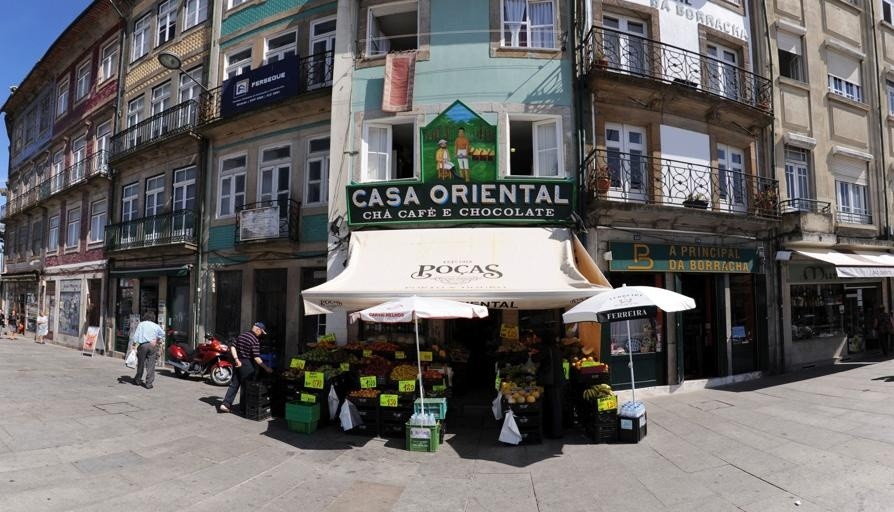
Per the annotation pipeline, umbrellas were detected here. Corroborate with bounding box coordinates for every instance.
[563,284,696,404]
[348,294,490,415]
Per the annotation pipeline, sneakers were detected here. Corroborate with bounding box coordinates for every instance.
[217,404,235,413]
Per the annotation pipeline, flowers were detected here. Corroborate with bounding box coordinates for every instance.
[756,192,778,203]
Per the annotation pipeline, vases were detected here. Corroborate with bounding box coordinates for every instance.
[762,201,774,209]
[597,178,611,193]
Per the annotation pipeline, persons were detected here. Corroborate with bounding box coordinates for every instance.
[36,311,48,344]
[455,127,470,182]
[873,305,894,357]
[219,322,272,413]
[0,309,5,337]
[6,310,19,340]
[527,328,563,439]
[436,139,464,181]
[131,311,165,388]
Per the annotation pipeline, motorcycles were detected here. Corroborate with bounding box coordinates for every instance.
[166,328,233,387]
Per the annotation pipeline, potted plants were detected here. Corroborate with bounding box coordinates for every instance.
[683,193,709,210]
[592,43,608,69]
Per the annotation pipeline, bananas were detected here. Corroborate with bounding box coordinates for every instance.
[582,384,613,400]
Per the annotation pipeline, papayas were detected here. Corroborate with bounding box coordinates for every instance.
[512,388,539,404]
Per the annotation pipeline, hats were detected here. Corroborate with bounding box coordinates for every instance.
[253,322,268,336]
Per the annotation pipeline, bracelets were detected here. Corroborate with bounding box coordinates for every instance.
[234,356,238,359]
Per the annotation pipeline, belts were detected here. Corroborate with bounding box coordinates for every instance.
[139,342,151,345]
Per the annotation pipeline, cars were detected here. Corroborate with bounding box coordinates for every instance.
[730,324,753,355]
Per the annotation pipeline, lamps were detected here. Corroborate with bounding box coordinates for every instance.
[158,52,214,97]
